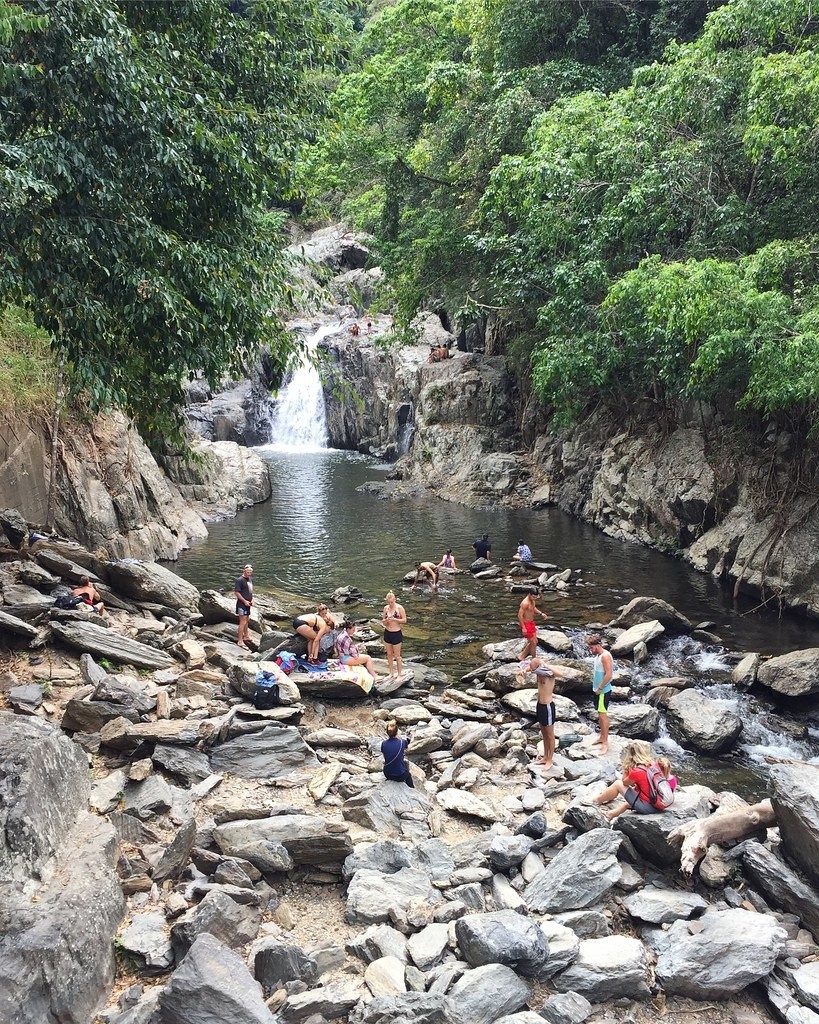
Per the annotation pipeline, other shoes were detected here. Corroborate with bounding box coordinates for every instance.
[307,657,322,666]
[374,675,384,683]
[596,807,613,823]
[579,797,602,808]
[28,657,44,665]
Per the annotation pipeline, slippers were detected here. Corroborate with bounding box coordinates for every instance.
[243,636,253,641]
[237,643,250,651]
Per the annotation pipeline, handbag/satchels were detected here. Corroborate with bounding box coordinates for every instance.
[318,648,327,662]
[275,657,293,674]
[54,595,84,610]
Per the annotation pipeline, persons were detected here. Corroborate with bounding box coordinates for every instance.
[315,604,338,630]
[518,587,547,661]
[437,549,457,569]
[512,539,532,562]
[73,575,104,616]
[382,590,407,680]
[294,614,334,665]
[380,723,414,788]
[658,758,677,792]
[410,561,439,592]
[592,742,674,822]
[587,636,614,755]
[530,658,561,766]
[472,534,491,560]
[336,621,384,681]
[234,564,253,650]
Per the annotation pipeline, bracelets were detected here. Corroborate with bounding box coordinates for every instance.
[599,688,602,690]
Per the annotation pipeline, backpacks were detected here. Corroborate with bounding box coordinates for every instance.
[252,671,280,710]
[637,762,675,811]
